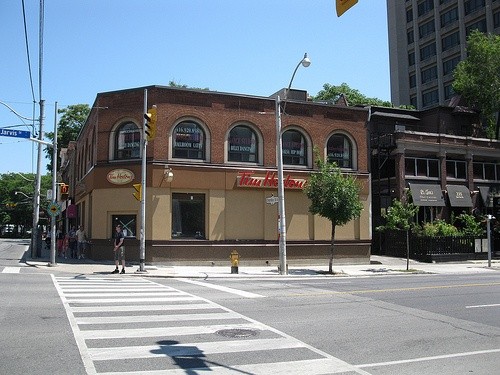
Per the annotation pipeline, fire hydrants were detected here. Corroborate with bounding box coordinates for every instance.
[229,249,240,274]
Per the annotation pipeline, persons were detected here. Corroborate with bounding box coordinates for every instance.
[76,224,88,260]
[46,226,62,249]
[112,224,125,273]
[68,225,78,259]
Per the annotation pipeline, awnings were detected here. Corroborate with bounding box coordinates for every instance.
[447,185,473,206]
[409,184,445,206]
[478,186,500,207]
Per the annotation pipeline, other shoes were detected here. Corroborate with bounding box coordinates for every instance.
[113,269,119,273]
[120,270,125,273]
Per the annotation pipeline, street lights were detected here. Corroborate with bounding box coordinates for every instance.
[275,53,312,273]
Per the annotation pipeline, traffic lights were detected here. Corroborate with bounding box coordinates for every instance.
[131,183,141,201]
[61,185,69,194]
[144,109,156,141]
